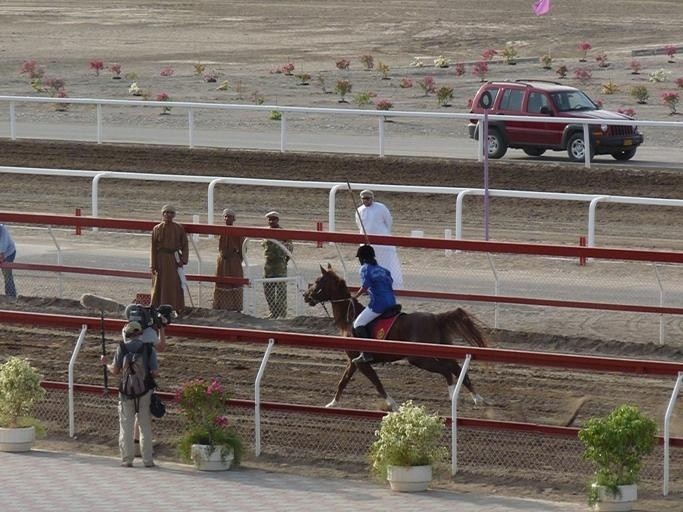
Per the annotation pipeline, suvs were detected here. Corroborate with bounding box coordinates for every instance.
[466,78,645,161]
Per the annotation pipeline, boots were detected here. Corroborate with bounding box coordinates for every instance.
[351,325,374,363]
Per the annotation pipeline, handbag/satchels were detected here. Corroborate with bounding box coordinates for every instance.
[151,395,165,417]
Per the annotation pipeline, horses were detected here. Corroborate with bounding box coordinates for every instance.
[302,262,495,413]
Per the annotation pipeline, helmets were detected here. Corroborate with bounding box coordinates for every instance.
[356,246,375,256]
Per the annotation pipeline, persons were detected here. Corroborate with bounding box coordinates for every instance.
[101,321,159,467]
[355,190,404,290]
[213,209,247,313]
[133,325,166,457]
[149,205,189,318]
[261,212,293,319]
[0,224,16,304]
[351,246,397,363]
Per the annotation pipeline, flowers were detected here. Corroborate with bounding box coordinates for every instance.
[176,376,234,443]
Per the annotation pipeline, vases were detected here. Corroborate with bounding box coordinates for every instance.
[592,483,638,502]
[387,465,432,492]
[191,442,233,472]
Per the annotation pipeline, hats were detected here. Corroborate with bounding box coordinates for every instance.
[265,212,279,218]
[124,322,143,338]
[360,191,374,199]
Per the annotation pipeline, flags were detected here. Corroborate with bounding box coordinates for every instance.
[531,0,551,17]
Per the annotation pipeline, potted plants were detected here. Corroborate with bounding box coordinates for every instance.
[579,405,657,508]
[0,356,47,454]
[374,397,447,493]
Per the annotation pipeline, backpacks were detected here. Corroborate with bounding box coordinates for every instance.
[120,341,148,396]
